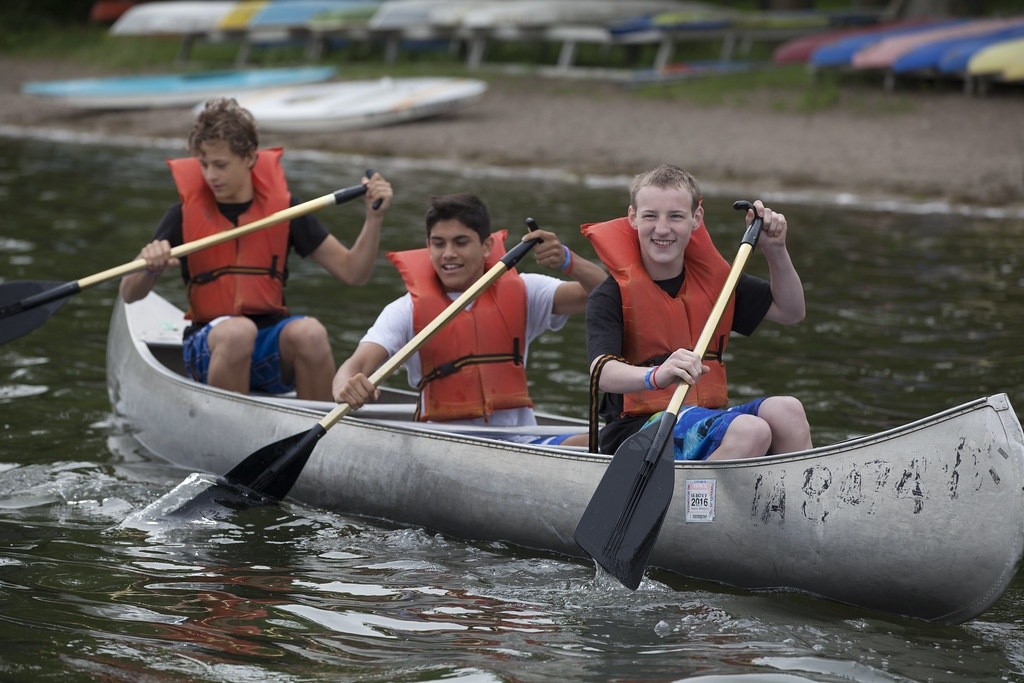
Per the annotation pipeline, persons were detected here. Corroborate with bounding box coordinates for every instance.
[331,195,609,451]
[579,165,813,461]
[120,95,393,402]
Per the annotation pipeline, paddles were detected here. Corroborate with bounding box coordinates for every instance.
[159,216,546,524]
[568,200,768,592]
[0,167,383,351]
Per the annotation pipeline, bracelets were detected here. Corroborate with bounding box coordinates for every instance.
[645,365,672,390]
[560,244,573,276]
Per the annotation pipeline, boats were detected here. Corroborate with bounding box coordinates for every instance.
[776,12,1024,87]
[106,279,1023,624]
[19,64,341,111]
[191,75,492,136]
